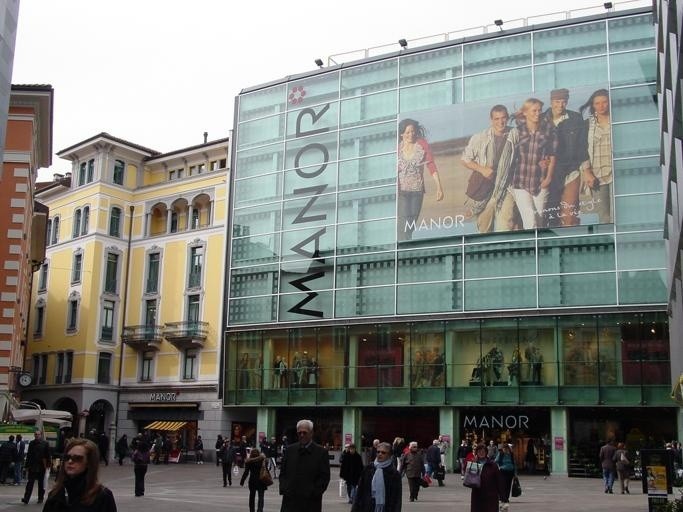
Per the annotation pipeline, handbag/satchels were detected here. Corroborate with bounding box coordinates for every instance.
[464,170,495,202]
[461,460,485,490]
[339,479,348,498]
[577,166,597,215]
[419,467,446,489]
[475,198,497,234]
[619,452,630,465]
[258,458,274,486]
[510,476,522,498]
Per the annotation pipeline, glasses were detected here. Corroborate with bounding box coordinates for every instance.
[61,453,85,463]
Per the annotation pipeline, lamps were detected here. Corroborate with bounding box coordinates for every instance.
[493,19,504,32]
[314,58,324,69]
[603,1,613,13]
[397,39,408,50]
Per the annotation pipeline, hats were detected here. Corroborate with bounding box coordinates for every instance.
[550,87,570,101]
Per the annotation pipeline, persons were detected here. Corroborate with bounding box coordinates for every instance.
[460,89,613,232]
[399,119,444,241]
